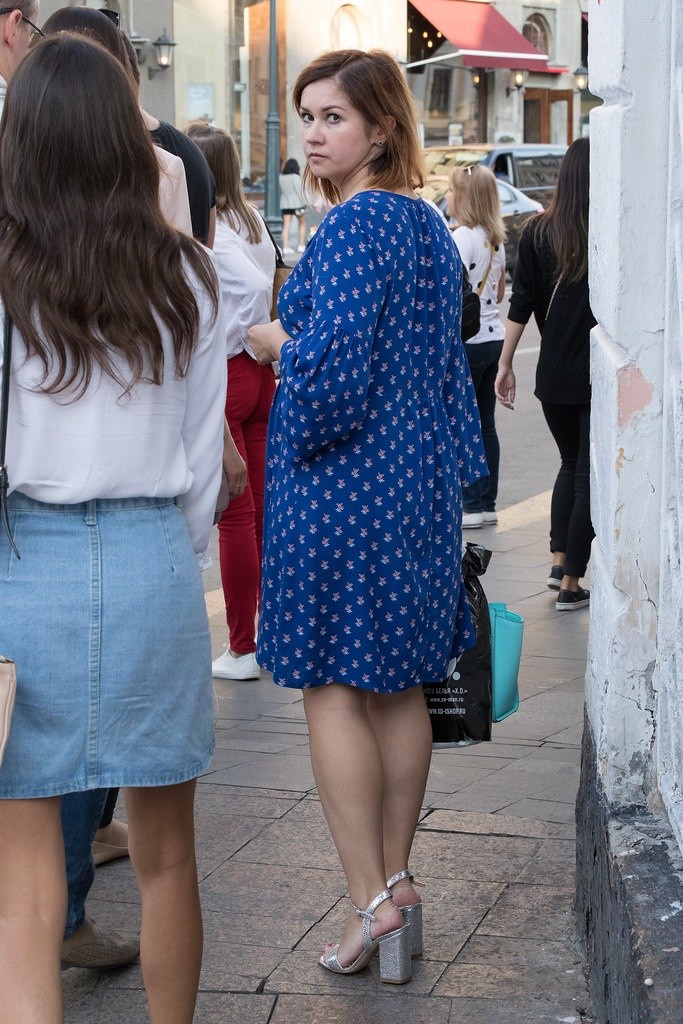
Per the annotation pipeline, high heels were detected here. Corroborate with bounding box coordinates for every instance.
[318,890,411,983]
[385,869,425,956]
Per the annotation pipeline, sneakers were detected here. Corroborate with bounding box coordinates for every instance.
[547,565,566,590]
[298,245,305,252]
[556,586,590,610]
[482,511,498,524]
[212,648,260,679]
[462,513,483,528]
[283,248,295,254]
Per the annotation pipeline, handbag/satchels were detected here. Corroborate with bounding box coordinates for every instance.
[0,655,17,767]
[421,542,492,749]
[252,206,294,321]
[488,603,523,723]
[460,283,482,342]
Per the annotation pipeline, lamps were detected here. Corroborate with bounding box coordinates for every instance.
[148,29,176,80]
[506,68,529,97]
[574,65,589,92]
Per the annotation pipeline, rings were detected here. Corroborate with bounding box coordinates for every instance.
[233,484,242,488]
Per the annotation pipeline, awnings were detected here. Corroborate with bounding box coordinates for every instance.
[407,0,550,71]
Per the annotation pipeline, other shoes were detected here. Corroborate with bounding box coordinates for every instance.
[91,818,129,865]
[58,914,140,970]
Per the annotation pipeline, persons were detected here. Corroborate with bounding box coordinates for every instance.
[0,27,227,1023]
[0,0,40,123]
[279,159,306,255]
[27,6,249,969]
[120,28,217,251]
[445,164,508,527]
[243,48,491,985]
[494,135,598,610]
[180,120,278,681]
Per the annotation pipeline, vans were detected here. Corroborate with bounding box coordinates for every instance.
[423,143,569,222]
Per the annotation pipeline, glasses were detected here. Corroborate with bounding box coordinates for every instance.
[97,9,121,33]
[0,8,47,49]
[463,164,475,178]
